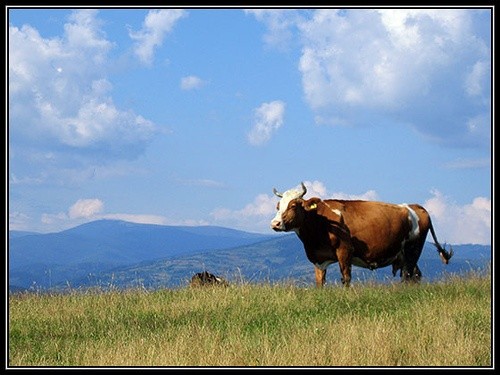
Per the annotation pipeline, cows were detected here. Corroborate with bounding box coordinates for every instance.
[192,272,228,287]
[271,182,454,289]
